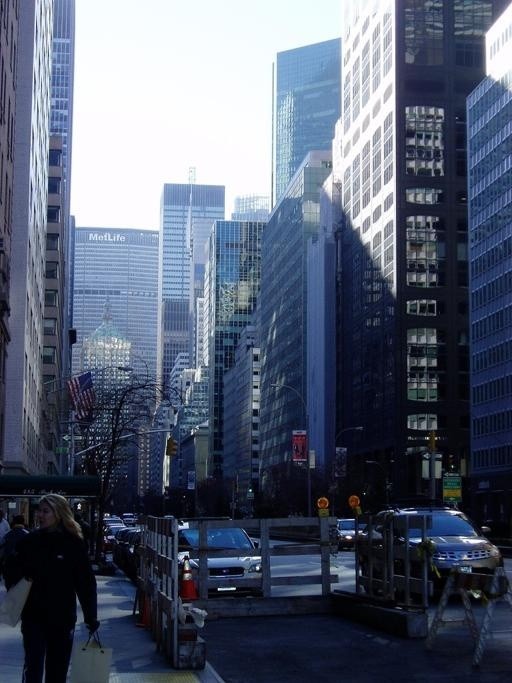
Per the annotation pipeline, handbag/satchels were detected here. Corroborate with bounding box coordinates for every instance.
[70,630,114,683]
[0,577,31,628]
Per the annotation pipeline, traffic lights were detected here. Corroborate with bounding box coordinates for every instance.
[167,435,179,457]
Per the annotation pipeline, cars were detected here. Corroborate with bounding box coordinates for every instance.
[102,513,262,595]
[330,506,504,596]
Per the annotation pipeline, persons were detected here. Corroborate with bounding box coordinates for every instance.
[0,494,100,683]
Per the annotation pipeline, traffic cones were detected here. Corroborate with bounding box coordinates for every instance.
[180,556,199,600]
[135,591,152,627]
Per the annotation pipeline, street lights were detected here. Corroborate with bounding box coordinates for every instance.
[335,427,364,442]
[269,382,311,517]
[71,365,134,474]
